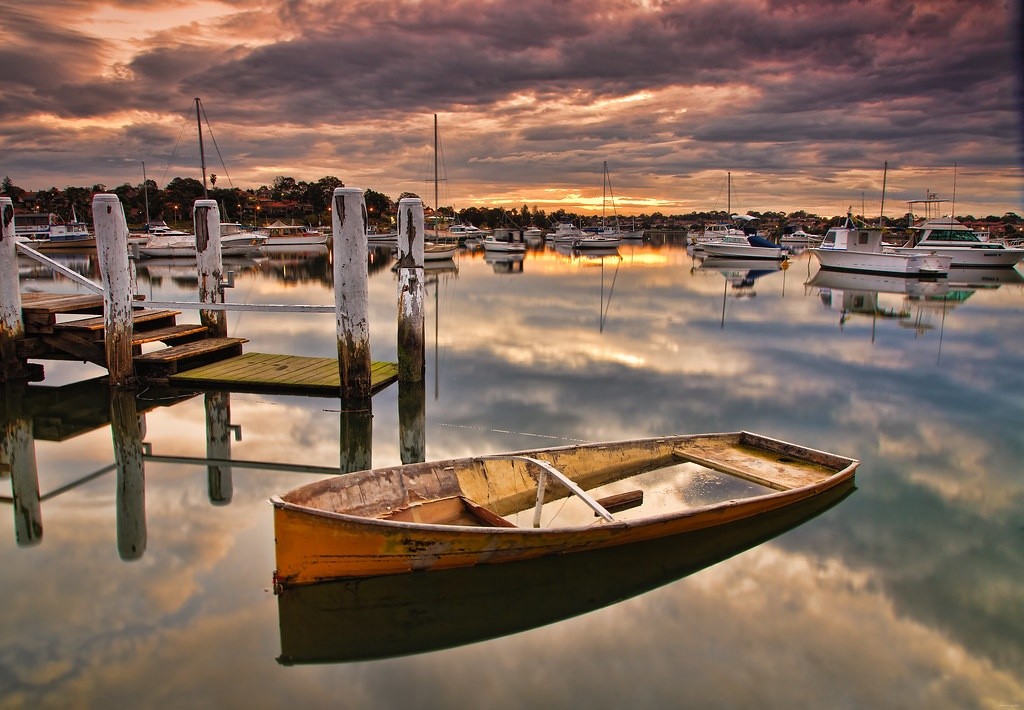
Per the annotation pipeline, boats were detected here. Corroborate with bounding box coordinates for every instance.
[326,206,400,242]
[899,267,1024,338]
[255,220,329,246]
[268,426,864,593]
[522,217,542,236]
[780,231,820,243]
[276,473,861,668]
[685,172,771,252]
[808,161,954,278]
[572,247,621,257]
[907,160,1024,268]
[482,250,526,275]
[424,215,469,246]
[572,235,621,249]
[127,161,191,244]
[804,270,950,344]
[480,235,527,252]
[261,245,329,269]
[464,225,492,240]
[142,256,255,310]
[580,160,652,239]
[13,204,97,255]
[546,213,621,242]
[696,256,784,330]
[699,215,789,261]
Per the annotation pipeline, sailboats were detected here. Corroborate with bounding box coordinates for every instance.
[390,260,459,401]
[138,95,267,257]
[392,114,459,261]
